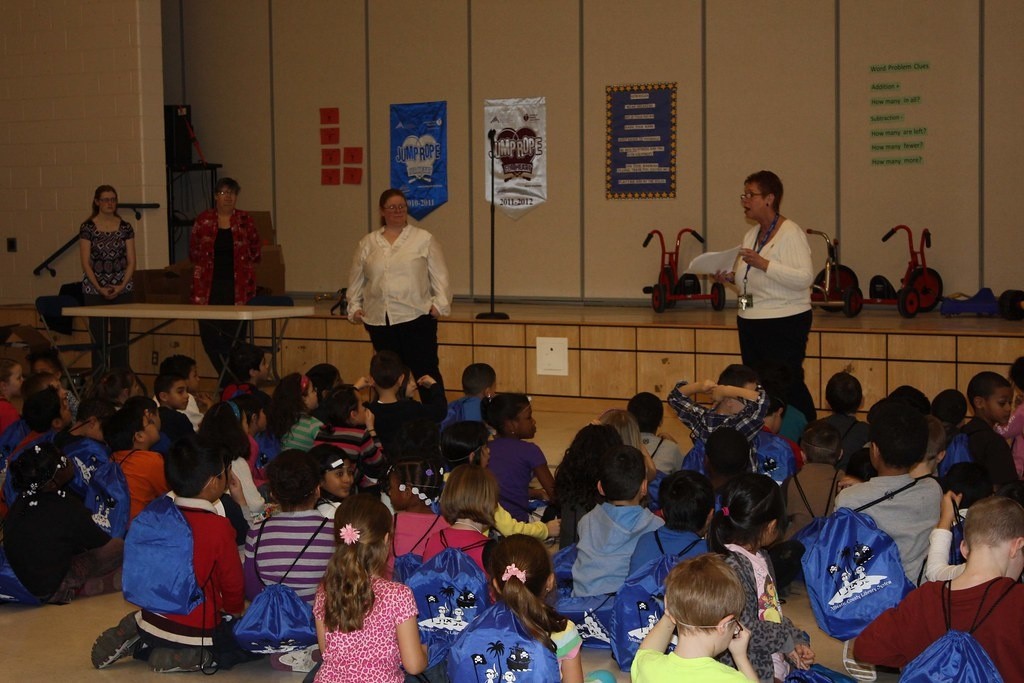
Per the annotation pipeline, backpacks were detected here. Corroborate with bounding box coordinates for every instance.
[121,496,217,616]
[447,601,561,683]
[800,481,927,642]
[85,449,140,539]
[681,411,708,476]
[0,539,41,605]
[407,531,492,670]
[553,543,578,588]
[555,589,619,649]
[232,517,329,652]
[4,430,61,505]
[610,530,704,672]
[392,513,441,582]
[785,663,859,683]
[938,426,990,477]
[643,468,665,511]
[898,578,1019,683]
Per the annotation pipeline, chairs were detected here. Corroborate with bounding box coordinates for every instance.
[36,281,99,402]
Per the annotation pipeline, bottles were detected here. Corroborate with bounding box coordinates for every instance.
[337,288,348,316]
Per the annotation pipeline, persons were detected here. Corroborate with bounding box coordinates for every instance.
[313,497,429,682]
[708,170,817,423]
[346,188,452,422]
[444,533,618,683]
[192,177,264,393]
[0,349,1024,683]
[80,185,136,404]
[630,551,761,682]
[853,494,1024,682]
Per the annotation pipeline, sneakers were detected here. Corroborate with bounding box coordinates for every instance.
[91,610,143,669]
[149,646,213,673]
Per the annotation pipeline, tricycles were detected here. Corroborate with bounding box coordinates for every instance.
[843,224,942,318]
[807,227,859,312]
[643,228,725,313]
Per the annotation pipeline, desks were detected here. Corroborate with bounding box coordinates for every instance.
[62,303,315,405]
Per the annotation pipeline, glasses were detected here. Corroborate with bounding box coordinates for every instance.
[99,197,117,203]
[384,204,407,209]
[218,190,236,196]
[740,192,767,200]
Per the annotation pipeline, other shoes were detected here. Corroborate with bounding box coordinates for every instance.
[843,636,877,681]
